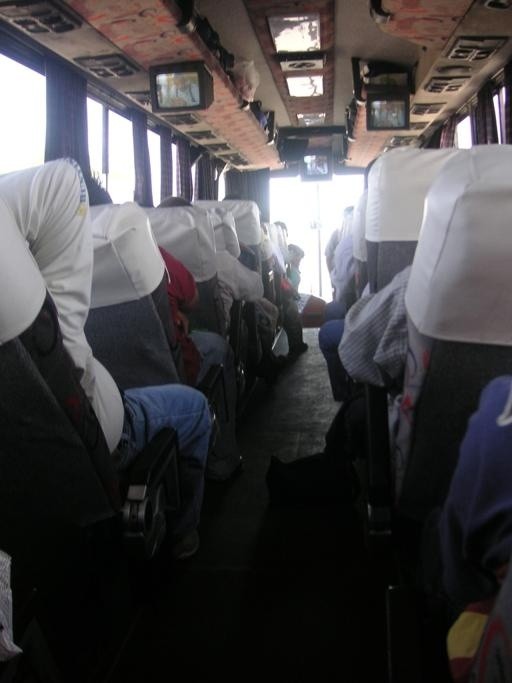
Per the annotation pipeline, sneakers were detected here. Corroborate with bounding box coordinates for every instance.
[175,530,200,559]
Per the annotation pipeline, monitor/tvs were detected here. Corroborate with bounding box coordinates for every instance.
[147,59,215,114]
[366,91,410,131]
[299,145,332,183]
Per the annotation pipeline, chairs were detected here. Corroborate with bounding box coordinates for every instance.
[192,199,262,281]
[147,202,247,372]
[1,197,183,577]
[209,207,242,260]
[351,188,366,298]
[83,200,224,432]
[366,147,465,291]
[373,144,511,512]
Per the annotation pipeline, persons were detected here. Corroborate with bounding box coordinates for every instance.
[283,244,304,296]
[436,374,512,604]
[317,156,380,402]
[0,155,211,560]
[161,73,199,106]
[78,164,244,453]
[259,222,309,358]
[337,261,415,389]
[154,195,271,411]
[329,230,360,302]
[323,206,356,302]
[214,223,288,371]
